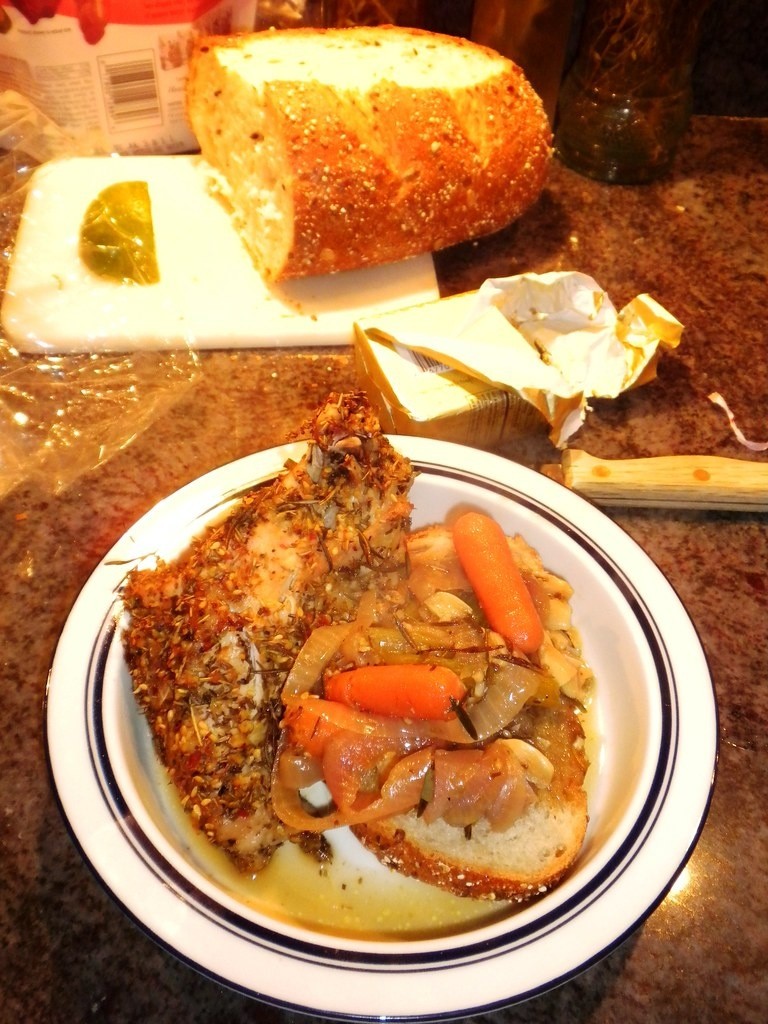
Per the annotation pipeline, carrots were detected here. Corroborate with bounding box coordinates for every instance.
[453,510,545,656]
[324,665,471,722]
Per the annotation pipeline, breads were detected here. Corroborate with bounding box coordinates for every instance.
[186,25,554,285]
[348,524,590,900]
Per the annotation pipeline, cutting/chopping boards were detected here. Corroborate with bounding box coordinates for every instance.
[0,155,440,355]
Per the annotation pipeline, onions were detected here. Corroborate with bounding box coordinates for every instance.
[270,565,555,834]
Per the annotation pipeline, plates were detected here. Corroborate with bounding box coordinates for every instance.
[48,434,721,1023]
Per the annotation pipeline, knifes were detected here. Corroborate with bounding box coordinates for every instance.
[524,449,768,513]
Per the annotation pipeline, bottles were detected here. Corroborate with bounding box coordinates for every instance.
[553,1,712,183]
